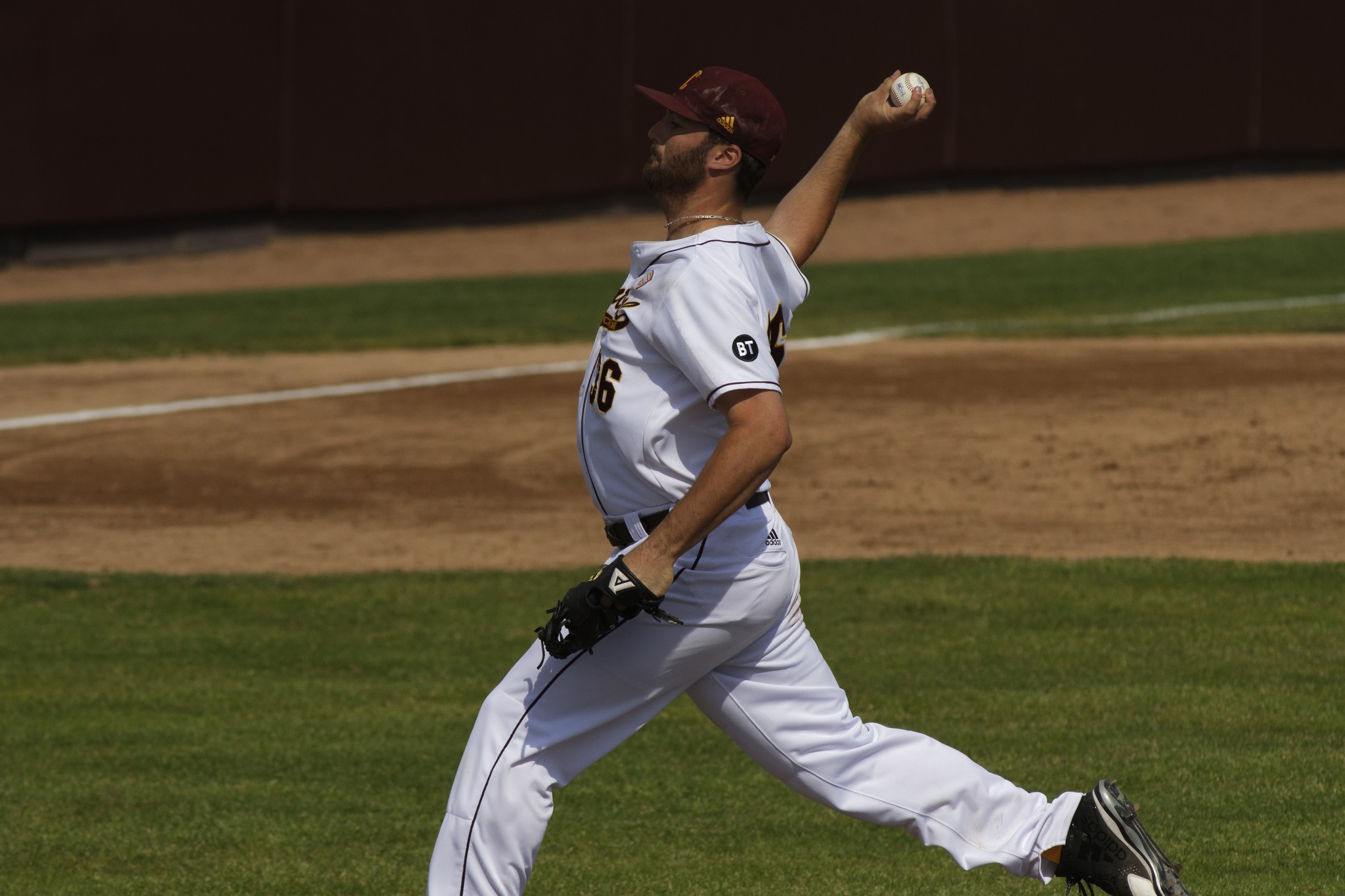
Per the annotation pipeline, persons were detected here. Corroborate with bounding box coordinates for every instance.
[426,65,1187,896]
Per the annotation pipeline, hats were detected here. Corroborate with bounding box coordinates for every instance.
[635,68,784,167]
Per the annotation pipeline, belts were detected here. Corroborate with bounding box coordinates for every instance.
[605,488,769,548]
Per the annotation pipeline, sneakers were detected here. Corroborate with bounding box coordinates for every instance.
[1053,779,1190,896]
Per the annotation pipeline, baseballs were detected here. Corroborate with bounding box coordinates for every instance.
[890,72,930,111]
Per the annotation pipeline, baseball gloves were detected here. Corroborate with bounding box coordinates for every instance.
[543,554,665,660]
